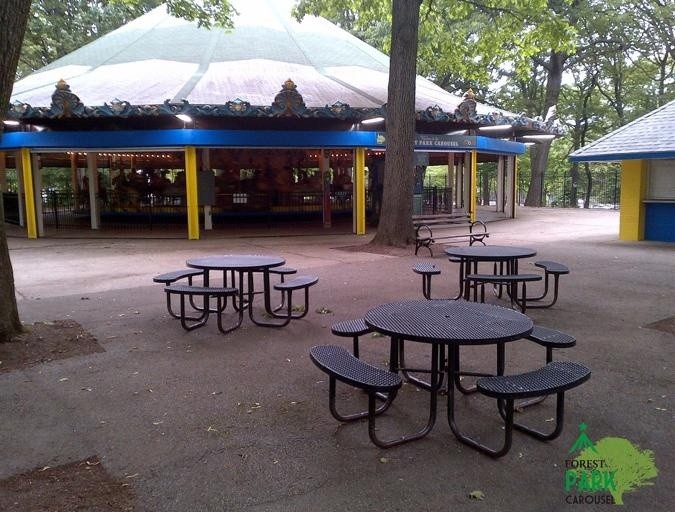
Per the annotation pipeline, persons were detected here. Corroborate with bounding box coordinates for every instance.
[81,163,383,212]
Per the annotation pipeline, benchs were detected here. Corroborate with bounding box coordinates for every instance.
[152,244,595,464]
[410,209,491,256]
